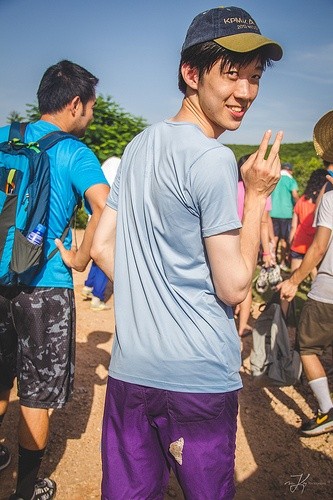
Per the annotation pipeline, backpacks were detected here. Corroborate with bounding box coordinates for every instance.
[0,121,81,289]
[249,291,302,387]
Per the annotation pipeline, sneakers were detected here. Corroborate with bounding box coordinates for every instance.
[298,407,333,435]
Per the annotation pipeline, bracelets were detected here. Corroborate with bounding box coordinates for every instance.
[263,252,270,256]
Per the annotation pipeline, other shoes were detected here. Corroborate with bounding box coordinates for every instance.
[0,446,10,470]
[80,289,91,298]
[90,302,110,311]
[268,265,280,284]
[9,478,55,500]
[255,266,268,293]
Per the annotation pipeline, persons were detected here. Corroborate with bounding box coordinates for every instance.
[276,111,332,435]
[80,155,125,311]
[233,154,333,338]
[89,6,283,500]
[0,60,111,500]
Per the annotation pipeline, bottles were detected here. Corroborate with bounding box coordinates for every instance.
[26,224,47,245]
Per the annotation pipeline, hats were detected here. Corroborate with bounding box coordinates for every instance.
[181,6,283,61]
[312,109,333,164]
